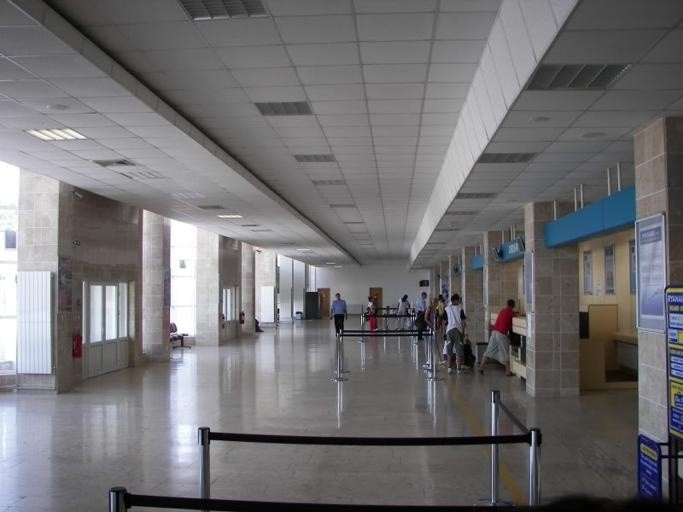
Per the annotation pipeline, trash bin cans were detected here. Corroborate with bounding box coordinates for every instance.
[296,312,302,320]
[476,342,489,364]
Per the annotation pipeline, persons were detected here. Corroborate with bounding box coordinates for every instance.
[329,293,348,336]
[396,291,469,375]
[366,296,379,330]
[477,298,521,376]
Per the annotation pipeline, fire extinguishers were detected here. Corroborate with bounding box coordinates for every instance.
[73,329,83,358]
[240,311,245,324]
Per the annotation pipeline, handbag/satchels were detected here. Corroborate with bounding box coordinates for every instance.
[464,343,475,363]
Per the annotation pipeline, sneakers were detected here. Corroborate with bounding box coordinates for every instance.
[478,369,483,373]
[439,361,462,374]
[506,372,514,376]
